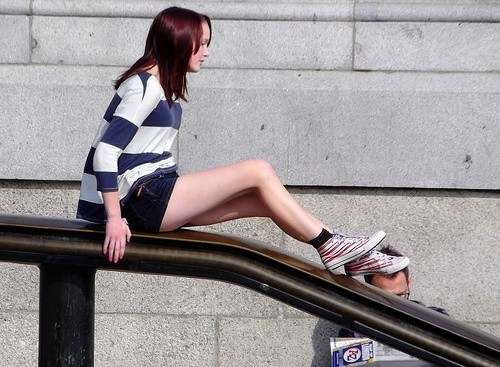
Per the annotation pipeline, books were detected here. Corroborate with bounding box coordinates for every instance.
[330,337,419,367]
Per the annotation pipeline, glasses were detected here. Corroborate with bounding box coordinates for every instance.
[396,287,410,300]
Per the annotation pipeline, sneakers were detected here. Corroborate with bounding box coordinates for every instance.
[317,230,386,271]
[344,249,410,276]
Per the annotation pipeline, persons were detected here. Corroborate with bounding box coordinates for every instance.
[76,7,410,277]
[338,244,450,338]
